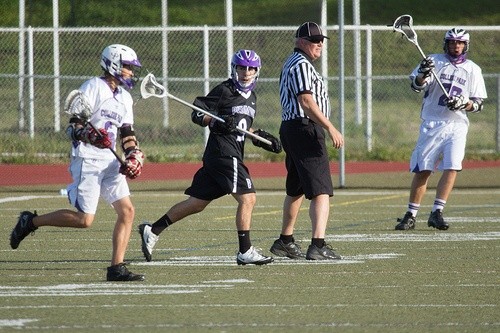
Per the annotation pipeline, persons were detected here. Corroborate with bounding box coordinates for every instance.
[395,28,487,229]
[9,43,145,282]
[269,23,344,261]
[139,48,280,265]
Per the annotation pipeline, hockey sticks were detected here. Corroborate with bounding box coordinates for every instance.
[139,74,272,145]
[64,89,125,168]
[393,14,449,98]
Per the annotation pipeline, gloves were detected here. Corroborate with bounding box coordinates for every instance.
[209,113,238,136]
[251,129,283,154]
[82,127,112,149]
[117,150,146,180]
[417,56,436,81]
[445,94,469,111]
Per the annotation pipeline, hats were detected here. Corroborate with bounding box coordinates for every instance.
[295,22,330,41]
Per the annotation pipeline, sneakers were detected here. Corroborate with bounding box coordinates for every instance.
[427,209,450,230]
[107,258,144,282]
[236,245,274,266]
[393,212,417,231]
[269,238,306,260]
[138,223,160,262]
[9,210,39,249]
[304,241,341,261]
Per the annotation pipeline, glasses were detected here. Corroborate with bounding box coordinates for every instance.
[304,38,324,44]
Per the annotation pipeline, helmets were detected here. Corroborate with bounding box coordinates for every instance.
[443,27,470,57]
[229,50,261,93]
[99,44,143,91]
